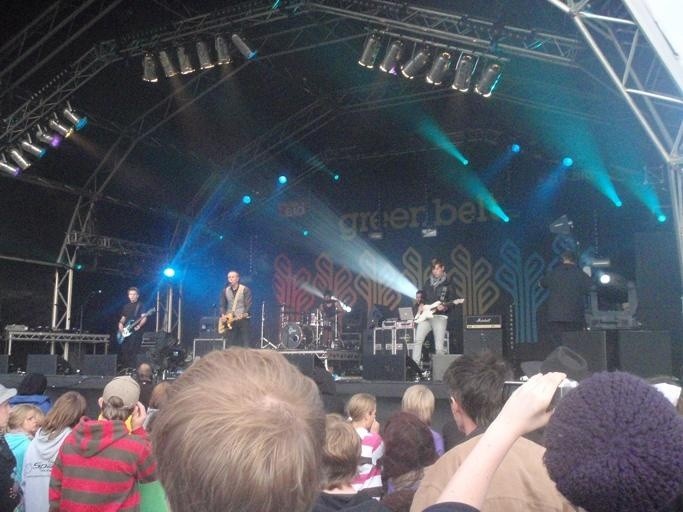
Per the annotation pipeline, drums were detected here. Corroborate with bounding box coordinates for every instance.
[280,324,314,349]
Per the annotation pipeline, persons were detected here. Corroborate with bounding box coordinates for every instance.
[534,250,585,343]
[1,347,682,512]
[116,286,148,369]
[414,287,427,314]
[319,290,344,347]
[409,257,456,366]
[216,270,252,350]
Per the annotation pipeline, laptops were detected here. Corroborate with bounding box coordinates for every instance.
[398,308,415,319]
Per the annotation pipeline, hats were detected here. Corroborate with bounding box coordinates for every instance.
[538,365,683,512]
[136,362,154,377]
[100,374,142,411]
[0,381,17,406]
[519,346,595,390]
[17,372,48,396]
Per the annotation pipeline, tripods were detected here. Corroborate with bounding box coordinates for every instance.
[304,309,328,350]
[256,301,278,350]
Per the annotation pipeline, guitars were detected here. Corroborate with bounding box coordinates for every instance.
[117,307,155,344]
[218,313,248,334]
[414,298,464,323]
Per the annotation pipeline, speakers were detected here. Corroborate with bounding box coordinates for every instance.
[461,330,503,363]
[278,353,326,378]
[26,353,70,374]
[0,355,17,373]
[633,233,679,332]
[82,354,127,376]
[561,330,617,373]
[614,328,682,379]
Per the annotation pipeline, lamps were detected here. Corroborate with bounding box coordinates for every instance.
[129,23,257,83]
[358,25,512,97]
[596,269,629,300]
[0,97,87,178]
